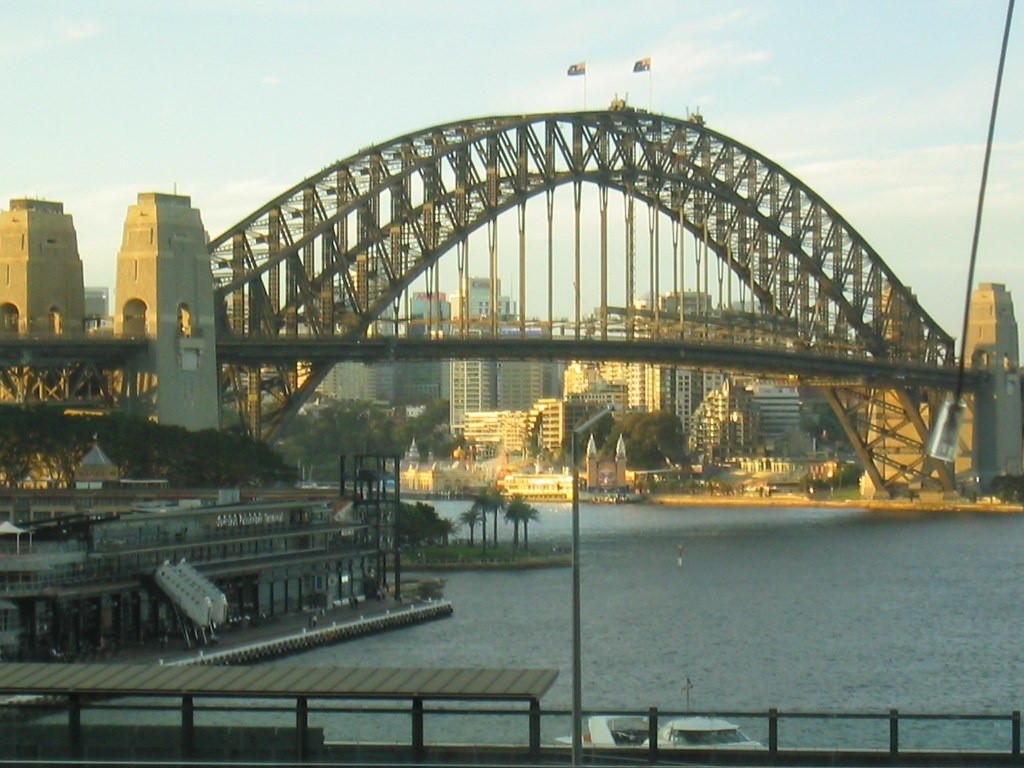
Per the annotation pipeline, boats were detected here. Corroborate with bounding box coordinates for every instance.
[555,715,764,750]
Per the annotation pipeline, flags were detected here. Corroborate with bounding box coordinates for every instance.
[633,58,650,72]
[568,62,585,75]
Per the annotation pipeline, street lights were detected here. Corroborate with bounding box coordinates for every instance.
[569,403,626,768]
